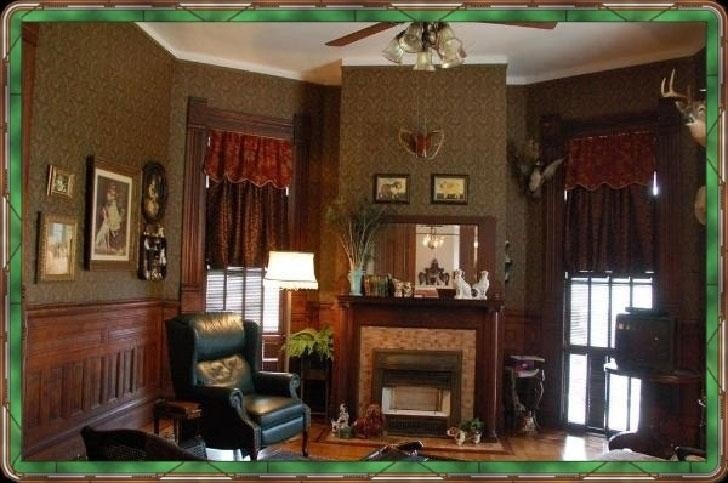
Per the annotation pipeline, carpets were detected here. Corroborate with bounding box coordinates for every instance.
[313,417,511,454]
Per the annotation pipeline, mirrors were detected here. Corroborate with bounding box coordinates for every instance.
[350,213,505,302]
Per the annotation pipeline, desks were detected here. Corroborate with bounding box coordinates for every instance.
[151,400,201,446]
[601,360,703,460]
[297,366,332,427]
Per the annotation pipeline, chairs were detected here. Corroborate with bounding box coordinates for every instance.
[79,420,427,461]
[504,366,549,430]
[161,303,312,460]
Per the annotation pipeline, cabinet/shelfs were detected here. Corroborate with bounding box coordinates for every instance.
[333,293,505,442]
[138,230,167,282]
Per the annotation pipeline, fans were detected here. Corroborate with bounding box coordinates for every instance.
[324,15,557,48]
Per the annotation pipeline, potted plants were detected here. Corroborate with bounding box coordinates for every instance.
[325,189,389,296]
[464,417,485,444]
[284,323,337,366]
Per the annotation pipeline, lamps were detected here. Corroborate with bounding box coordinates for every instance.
[382,19,468,71]
[258,244,318,372]
[419,222,444,250]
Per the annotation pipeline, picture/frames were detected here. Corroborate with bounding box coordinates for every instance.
[372,172,412,202]
[431,172,469,204]
[44,160,76,200]
[34,207,78,284]
[85,152,140,273]
